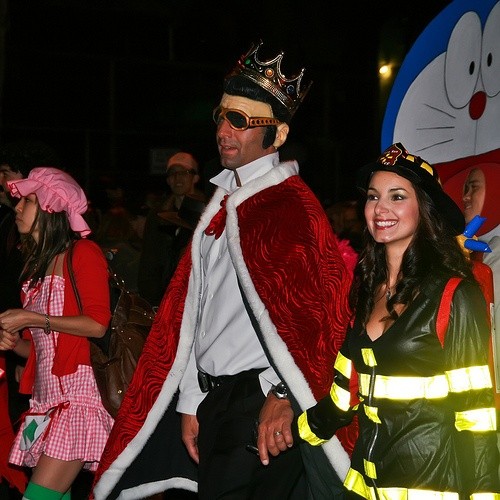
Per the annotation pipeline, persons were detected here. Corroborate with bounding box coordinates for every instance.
[0,166,111,500]
[455,163,500,415]
[317,172,370,248]
[255,138,500,500]
[172,71,352,500]
[0,145,208,313]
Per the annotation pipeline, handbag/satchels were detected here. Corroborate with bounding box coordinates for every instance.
[67,237,159,420]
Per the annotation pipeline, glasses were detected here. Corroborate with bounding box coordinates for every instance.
[213,105,280,131]
[167,171,192,176]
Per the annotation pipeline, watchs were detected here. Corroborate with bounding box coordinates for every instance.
[267,381,291,399]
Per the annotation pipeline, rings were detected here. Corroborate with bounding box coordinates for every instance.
[274,431,281,436]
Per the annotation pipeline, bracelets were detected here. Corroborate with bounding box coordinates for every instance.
[44,313,51,335]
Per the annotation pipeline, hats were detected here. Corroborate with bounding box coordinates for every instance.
[7,167,91,238]
[361,142,465,236]
[166,153,198,173]
